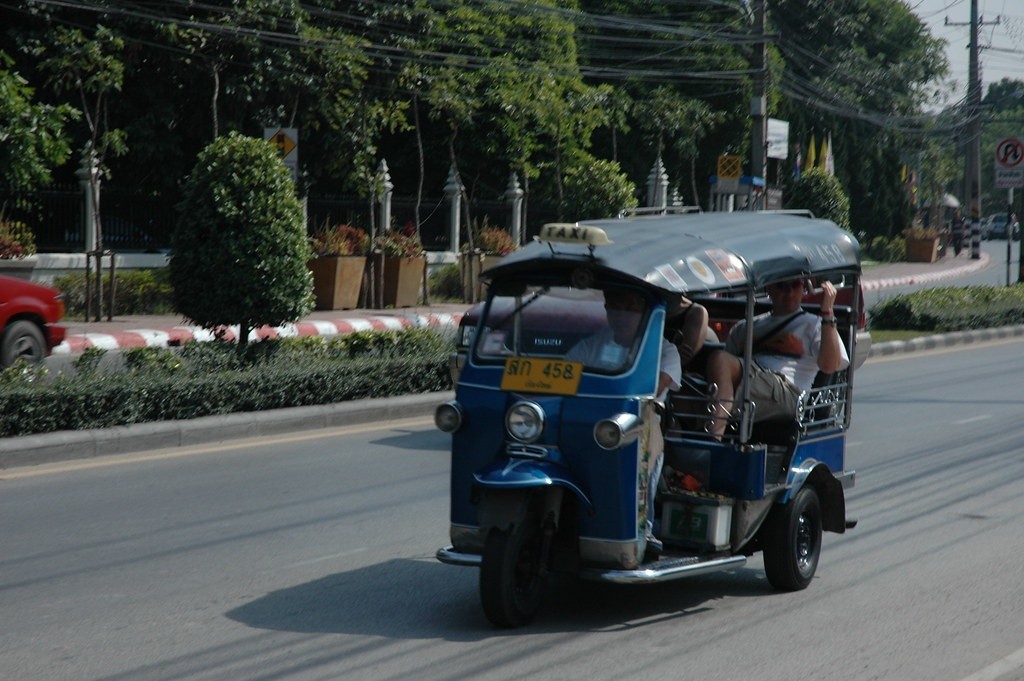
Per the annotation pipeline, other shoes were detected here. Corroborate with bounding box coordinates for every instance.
[646,530,663,551]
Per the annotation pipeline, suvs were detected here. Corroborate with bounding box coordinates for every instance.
[983,211,1022,240]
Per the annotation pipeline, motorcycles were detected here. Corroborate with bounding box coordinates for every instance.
[433,210,859,631]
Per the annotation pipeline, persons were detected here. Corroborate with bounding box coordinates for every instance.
[562,267,849,548]
[949,209,967,256]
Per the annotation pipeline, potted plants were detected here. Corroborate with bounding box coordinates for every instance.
[0,239,37,282]
[457,228,519,304]
[903,227,940,264]
[372,223,425,310]
[309,226,366,312]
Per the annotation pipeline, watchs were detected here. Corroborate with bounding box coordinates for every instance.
[820,317,838,327]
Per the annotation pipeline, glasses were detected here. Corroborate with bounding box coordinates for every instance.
[605,303,642,313]
[768,281,804,290]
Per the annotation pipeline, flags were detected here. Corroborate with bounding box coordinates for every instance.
[794,131,834,181]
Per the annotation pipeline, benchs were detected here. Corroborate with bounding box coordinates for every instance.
[688,298,851,445]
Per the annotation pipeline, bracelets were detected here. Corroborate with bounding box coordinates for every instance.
[820,309,833,316]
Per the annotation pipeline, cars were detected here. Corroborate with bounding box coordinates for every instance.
[0,274,71,366]
